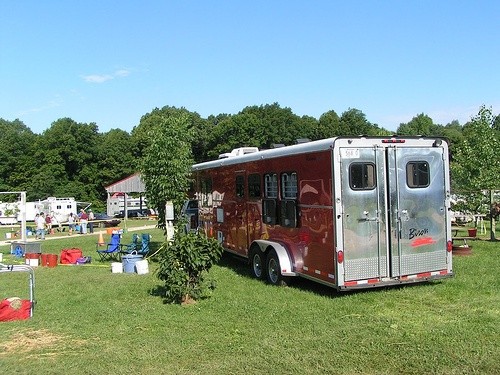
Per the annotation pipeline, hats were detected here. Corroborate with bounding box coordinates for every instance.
[88,209,92,211]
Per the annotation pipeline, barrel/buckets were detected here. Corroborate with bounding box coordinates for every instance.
[121,254,134,273]
[468,229,476,237]
[111,262,123,273]
[0,252,3,263]
[136,259,149,274]
[41,253,50,266]
[29,254,40,266]
[76,225,80,231]
[25,253,32,264]
[127,254,143,273]
[47,254,58,266]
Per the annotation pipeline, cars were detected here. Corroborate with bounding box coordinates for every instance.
[87,213,121,229]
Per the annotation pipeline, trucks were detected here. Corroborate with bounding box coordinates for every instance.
[189,133,454,293]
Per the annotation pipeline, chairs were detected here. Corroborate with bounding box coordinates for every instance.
[96,234,150,263]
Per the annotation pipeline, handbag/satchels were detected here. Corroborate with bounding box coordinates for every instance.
[0,296,33,322]
[60,248,82,264]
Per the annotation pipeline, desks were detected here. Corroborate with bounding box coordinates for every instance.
[452,228,465,244]
[11,242,41,255]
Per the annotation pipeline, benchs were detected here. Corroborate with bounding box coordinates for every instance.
[451,237,477,244]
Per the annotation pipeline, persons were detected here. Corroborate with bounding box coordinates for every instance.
[35,212,47,240]
[45,214,53,234]
[68,212,75,236]
[76,208,88,226]
[88,209,94,234]
